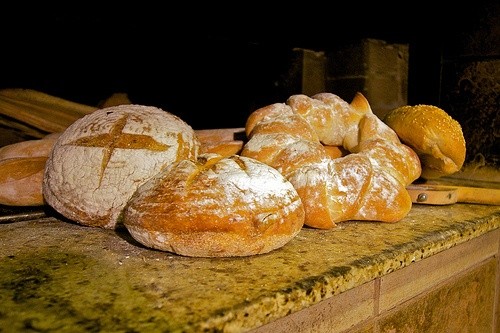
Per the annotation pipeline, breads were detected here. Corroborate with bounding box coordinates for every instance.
[0,89,467,258]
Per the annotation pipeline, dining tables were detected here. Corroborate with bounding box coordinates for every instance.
[0,176,500,333]
